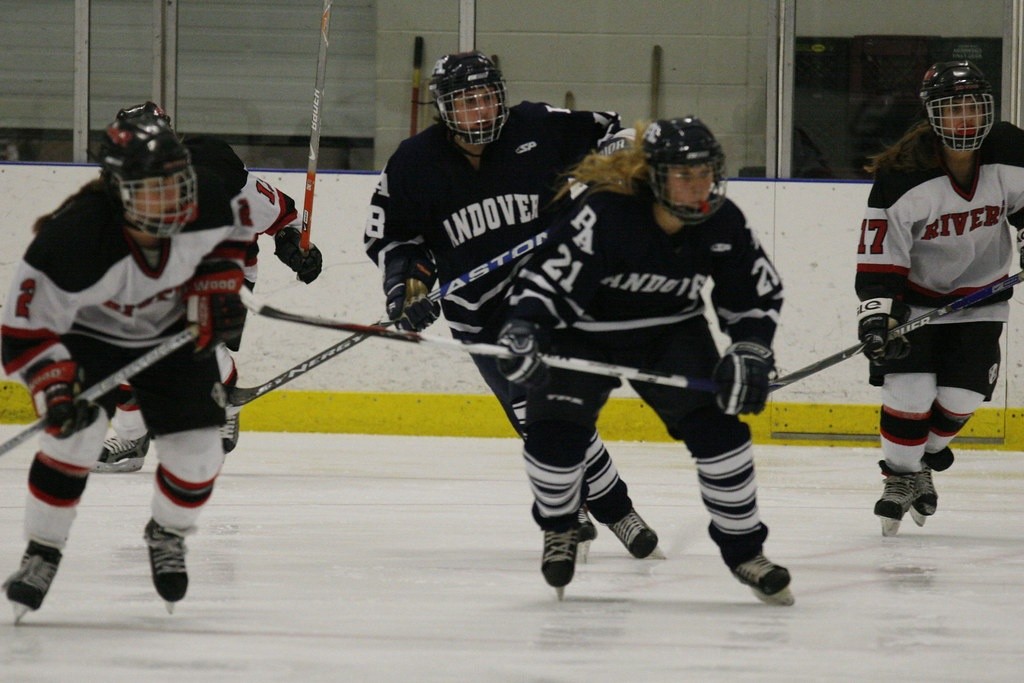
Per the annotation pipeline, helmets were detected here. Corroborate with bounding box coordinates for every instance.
[918,58,995,151]
[99,100,198,240]
[642,117,729,222]
[429,50,510,146]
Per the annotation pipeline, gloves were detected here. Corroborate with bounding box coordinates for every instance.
[42,381,100,441]
[492,320,551,394]
[273,227,324,285]
[378,278,444,335]
[713,346,778,421]
[1015,229,1024,269]
[857,317,911,369]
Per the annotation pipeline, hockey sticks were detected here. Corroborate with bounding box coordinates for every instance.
[234,279,727,393]
[0,328,200,463]
[223,224,566,404]
[296,0,337,256]
[769,272,1024,390]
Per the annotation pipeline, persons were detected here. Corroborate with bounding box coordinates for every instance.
[364,51,666,566]
[497,115,797,607]
[92,341,239,474]
[855,62,1024,536]
[0,101,323,625]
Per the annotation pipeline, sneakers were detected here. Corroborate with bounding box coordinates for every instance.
[542,529,578,600]
[872,458,916,539]
[142,518,198,615]
[2,539,63,626]
[576,507,599,563]
[910,463,938,528]
[219,405,241,455]
[722,551,795,608]
[91,434,151,474]
[610,507,667,560]
